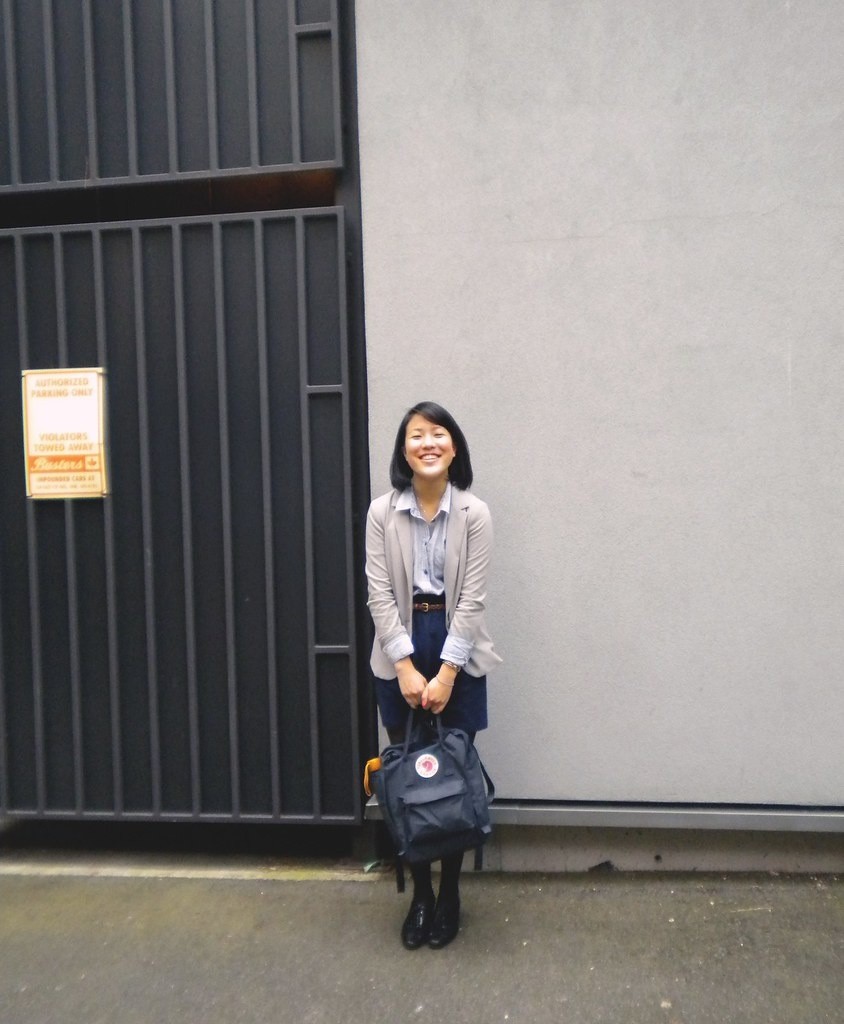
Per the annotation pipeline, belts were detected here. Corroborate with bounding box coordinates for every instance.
[413,603,445,612]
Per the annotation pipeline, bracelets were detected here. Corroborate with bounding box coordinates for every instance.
[442,659,458,673]
[436,675,454,687]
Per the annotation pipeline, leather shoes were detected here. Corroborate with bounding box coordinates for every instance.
[401,894,436,949]
[428,894,460,949]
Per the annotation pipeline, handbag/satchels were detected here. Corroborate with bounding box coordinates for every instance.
[363,702,494,867]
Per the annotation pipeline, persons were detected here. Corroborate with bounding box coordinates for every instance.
[364,402,503,950]
